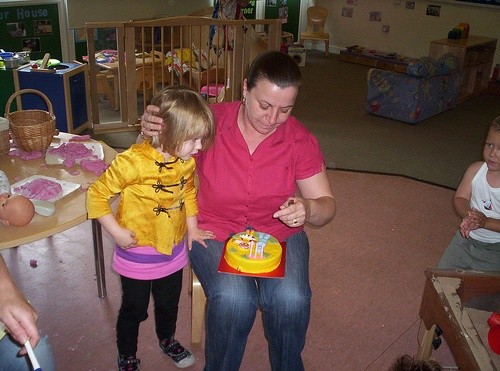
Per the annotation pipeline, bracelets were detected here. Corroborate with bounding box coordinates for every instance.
[140,133,150,141]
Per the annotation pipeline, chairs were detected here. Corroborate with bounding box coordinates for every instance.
[299,6,329,56]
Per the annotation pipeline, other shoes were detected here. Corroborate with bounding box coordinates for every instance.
[118,354,142,371]
[159,338,195,369]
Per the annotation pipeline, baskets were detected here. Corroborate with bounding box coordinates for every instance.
[0,117,10,157]
[4,89,56,153]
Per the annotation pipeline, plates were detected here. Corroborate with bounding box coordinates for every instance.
[9,174,81,203]
[45,142,104,164]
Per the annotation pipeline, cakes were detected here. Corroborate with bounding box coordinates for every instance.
[224,228,283,274]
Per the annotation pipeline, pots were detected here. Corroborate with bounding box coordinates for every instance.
[0,48,12,59]
[13,51,31,64]
[0,58,20,69]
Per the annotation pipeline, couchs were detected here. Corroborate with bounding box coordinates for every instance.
[366,54,464,124]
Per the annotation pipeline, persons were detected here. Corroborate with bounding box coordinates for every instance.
[135,52,337,370]
[86,83,217,371]
[0,169,35,226]
[433,115,500,350]
[0,248,54,371]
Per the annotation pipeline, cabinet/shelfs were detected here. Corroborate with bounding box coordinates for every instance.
[430,34,498,104]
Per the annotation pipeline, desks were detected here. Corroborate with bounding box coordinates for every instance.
[0,61,94,135]
[415,268,500,371]
[82,52,169,110]
[0,130,121,298]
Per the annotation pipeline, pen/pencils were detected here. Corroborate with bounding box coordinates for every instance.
[23,335,42,371]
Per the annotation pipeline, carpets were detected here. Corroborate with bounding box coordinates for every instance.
[0,168,462,371]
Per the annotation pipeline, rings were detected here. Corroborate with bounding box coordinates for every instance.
[293,218,297,224]
[141,131,144,137]
